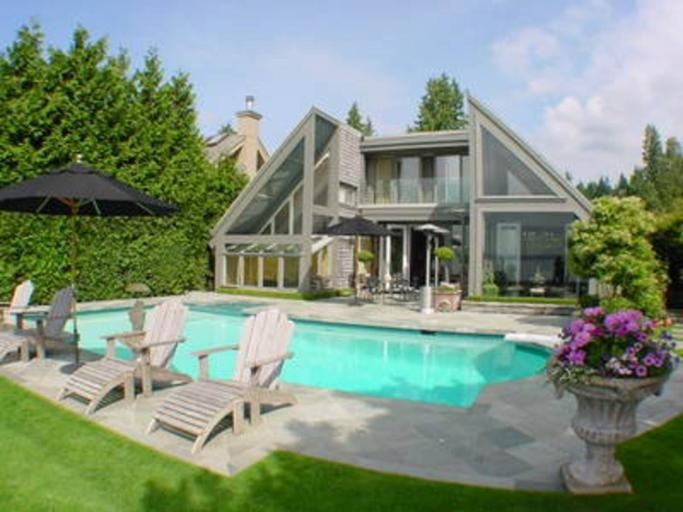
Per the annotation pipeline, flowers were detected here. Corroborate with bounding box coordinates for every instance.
[534,306,683,397]
[438,280,461,292]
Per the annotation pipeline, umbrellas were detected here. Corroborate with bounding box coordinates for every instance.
[1,154,184,368]
[312,213,401,299]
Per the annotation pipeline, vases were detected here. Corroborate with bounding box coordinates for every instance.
[432,289,463,314]
